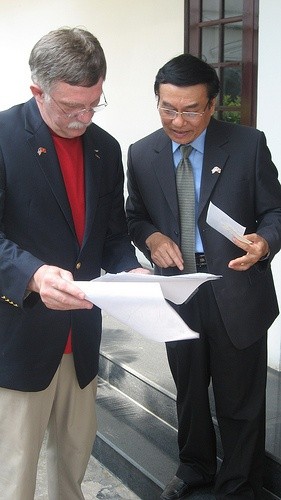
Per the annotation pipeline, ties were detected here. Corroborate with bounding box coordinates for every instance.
[176,144,196,304]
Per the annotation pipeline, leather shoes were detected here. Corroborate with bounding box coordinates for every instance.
[160,474,201,500]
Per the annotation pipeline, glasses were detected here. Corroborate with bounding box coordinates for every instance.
[44,91,107,119]
[157,96,213,121]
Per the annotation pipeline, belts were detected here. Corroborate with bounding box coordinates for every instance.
[194,254,206,266]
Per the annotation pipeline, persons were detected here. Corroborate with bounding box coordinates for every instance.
[0,26,151,500]
[126,53,281,500]
